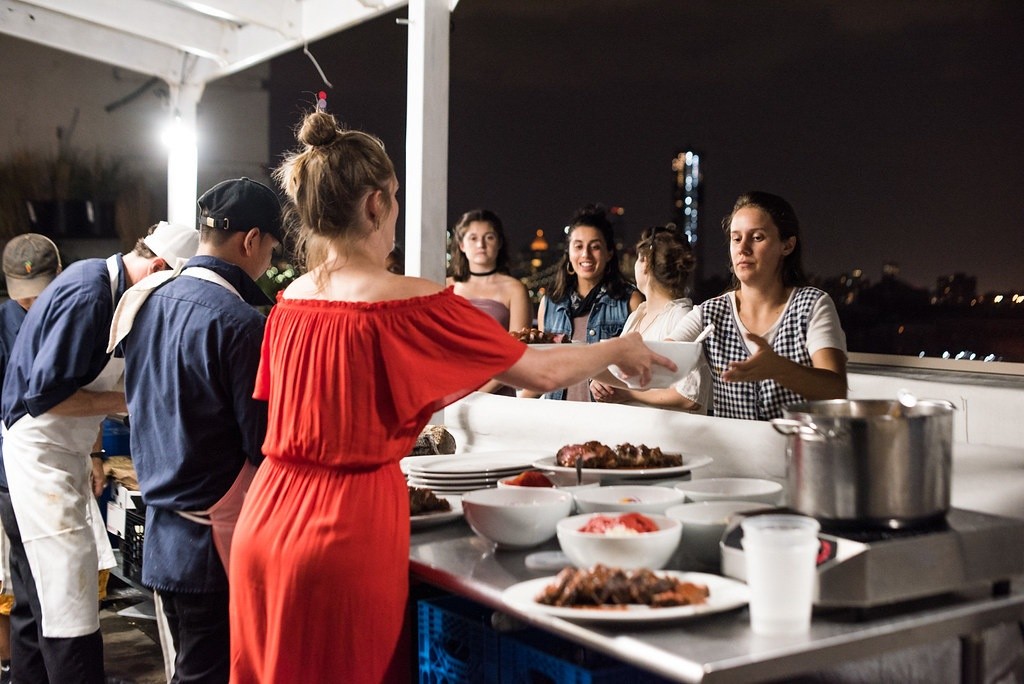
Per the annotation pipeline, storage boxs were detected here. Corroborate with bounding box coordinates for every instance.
[414,598,642,683]
[110,483,142,509]
[123,506,153,594]
[105,501,127,540]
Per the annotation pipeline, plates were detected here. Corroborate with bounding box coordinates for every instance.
[406,492,464,529]
[500,569,748,623]
[403,447,558,495]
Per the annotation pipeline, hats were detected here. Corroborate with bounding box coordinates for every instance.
[2,234,60,299]
[197,176,285,248]
[147,220,200,267]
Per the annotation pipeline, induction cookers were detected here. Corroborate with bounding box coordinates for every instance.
[717,504,1024,624]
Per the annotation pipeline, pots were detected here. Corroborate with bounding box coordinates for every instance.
[768,398,957,535]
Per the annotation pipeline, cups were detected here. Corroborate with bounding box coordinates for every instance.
[740,515,821,635]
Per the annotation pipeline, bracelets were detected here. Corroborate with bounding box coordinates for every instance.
[90,452,105,460]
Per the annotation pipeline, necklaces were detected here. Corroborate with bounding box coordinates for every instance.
[469,268,497,276]
[633,312,659,336]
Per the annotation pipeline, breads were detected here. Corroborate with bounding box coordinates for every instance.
[408,425,456,456]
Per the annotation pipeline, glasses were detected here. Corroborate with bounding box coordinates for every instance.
[648,224,689,255]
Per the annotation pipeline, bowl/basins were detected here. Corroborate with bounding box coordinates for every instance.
[530,455,713,488]
[526,340,583,350]
[556,511,684,570]
[600,336,702,389]
[675,476,782,503]
[665,500,775,563]
[460,487,575,550]
[573,483,686,516]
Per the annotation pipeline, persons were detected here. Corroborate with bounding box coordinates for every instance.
[589,225,715,416]
[446,209,533,396]
[107,177,288,684]
[516,202,646,402]
[657,190,849,422]
[227,109,678,684]
[0,221,201,684]
